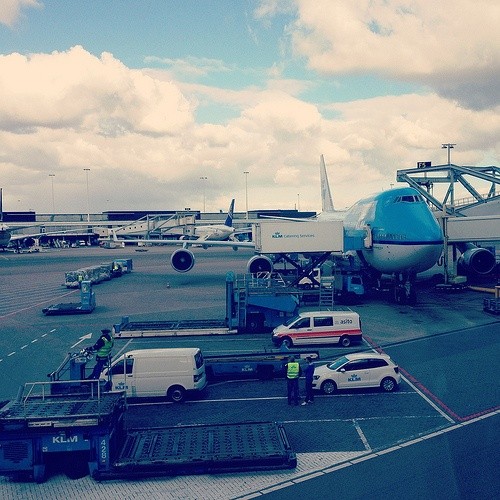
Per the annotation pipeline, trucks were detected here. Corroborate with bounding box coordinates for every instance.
[299,268,365,300]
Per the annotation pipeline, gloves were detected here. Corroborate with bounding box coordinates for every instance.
[89,347,92,351]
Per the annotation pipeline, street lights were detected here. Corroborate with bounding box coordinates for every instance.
[200,176,208,213]
[84,168,91,222]
[49,174,55,213]
[243,171,249,219]
[441,144,456,205]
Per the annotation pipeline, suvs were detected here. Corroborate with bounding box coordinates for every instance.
[59,240,69,248]
[311,353,403,395]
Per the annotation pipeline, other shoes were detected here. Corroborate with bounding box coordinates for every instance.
[301,401,308,406]
[288,403,291,405]
[294,402,299,406]
[308,400,313,403]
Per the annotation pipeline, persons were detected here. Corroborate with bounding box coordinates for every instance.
[301,356,315,406]
[285,356,302,405]
[78,273,84,288]
[84,328,114,386]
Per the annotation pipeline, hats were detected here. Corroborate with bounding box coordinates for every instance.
[101,328,111,333]
[290,356,295,361]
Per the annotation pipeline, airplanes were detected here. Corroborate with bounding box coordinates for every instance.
[113,153,496,305]
[0,187,44,252]
[162,198,236,250]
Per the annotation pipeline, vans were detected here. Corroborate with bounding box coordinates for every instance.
[271,311,362,349]
[79,240,86,246]
[99,348,208,403]
